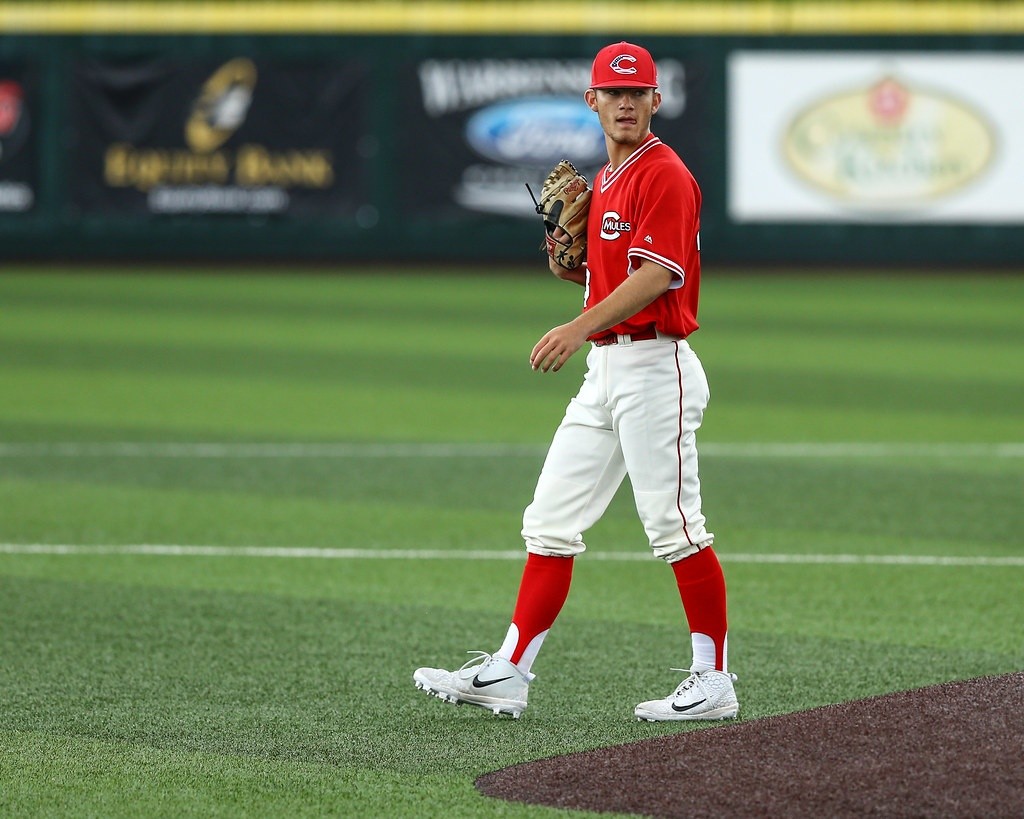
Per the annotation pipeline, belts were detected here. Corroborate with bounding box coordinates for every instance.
[594,330,657,347]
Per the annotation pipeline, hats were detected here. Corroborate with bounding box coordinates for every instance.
[589,41,660,89]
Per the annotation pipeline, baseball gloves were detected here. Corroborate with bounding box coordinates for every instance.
[524,155,593,271]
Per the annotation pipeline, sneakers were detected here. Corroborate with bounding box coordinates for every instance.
[635,665,739,723]
[413,651,529,719]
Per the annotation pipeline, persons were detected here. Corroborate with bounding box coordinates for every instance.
[415,41,739,723]
